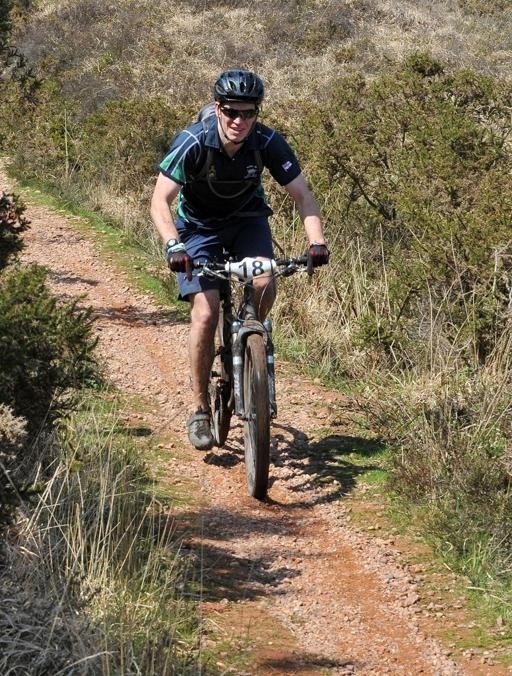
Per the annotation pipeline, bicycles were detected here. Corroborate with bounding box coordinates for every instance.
[189,254,308,500]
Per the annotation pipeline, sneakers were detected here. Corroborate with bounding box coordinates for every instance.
[185,405,214,451]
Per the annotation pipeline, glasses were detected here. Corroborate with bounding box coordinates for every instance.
[220,104,260,119]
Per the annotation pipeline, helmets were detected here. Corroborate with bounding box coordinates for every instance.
[213,69,265,106]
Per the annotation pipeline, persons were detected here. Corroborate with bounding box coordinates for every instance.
[149,66,325,450]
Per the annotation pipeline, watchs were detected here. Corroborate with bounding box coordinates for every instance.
[160,239,182,253]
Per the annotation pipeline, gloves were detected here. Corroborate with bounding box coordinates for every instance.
[163,239,194,282]
[304,240,331,276]
